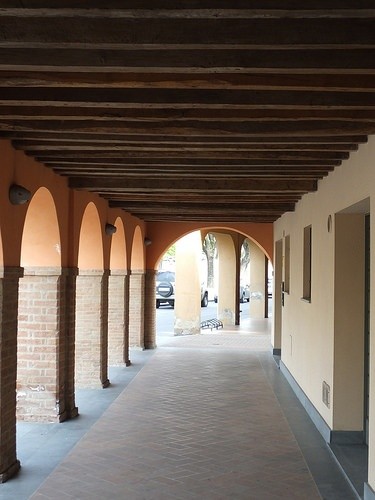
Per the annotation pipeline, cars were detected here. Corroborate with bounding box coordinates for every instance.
[213,282,250,304]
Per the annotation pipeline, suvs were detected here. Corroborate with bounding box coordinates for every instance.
[155,269,208,308]
